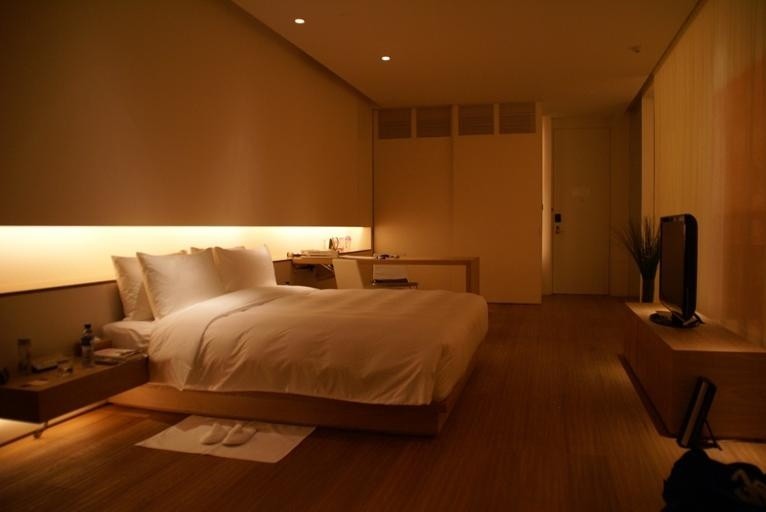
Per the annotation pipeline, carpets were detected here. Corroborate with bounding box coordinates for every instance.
[128,413,317,466]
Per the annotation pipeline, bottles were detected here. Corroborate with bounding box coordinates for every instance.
[16,336,32,375]
[329,237,351,251]
[80,321,95,368]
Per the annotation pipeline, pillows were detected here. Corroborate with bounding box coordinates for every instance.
[108,249,190,322]
[134,245,227,324]
[212,242,278,296]
[188,245,245,259]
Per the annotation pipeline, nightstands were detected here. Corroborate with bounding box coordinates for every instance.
[1,279,148,440]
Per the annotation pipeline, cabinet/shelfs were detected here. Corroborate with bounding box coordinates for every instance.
[619,301,766,442]
[289,245,482,297]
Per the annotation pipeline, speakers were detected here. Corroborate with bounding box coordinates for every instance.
[677,377,717,447]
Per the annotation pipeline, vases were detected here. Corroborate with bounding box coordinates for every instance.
[635,257,657,303]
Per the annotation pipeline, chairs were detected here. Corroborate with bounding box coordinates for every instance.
[330,254,420,290]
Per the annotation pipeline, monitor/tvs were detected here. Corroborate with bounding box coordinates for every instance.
[650,214,697,328]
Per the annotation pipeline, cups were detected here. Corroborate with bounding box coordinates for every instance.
[55,357,75,376]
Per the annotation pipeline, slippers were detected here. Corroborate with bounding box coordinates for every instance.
[222,424,256,447]
[200,422,228,445]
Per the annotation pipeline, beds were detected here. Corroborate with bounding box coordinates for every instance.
[98,273,492,438]
